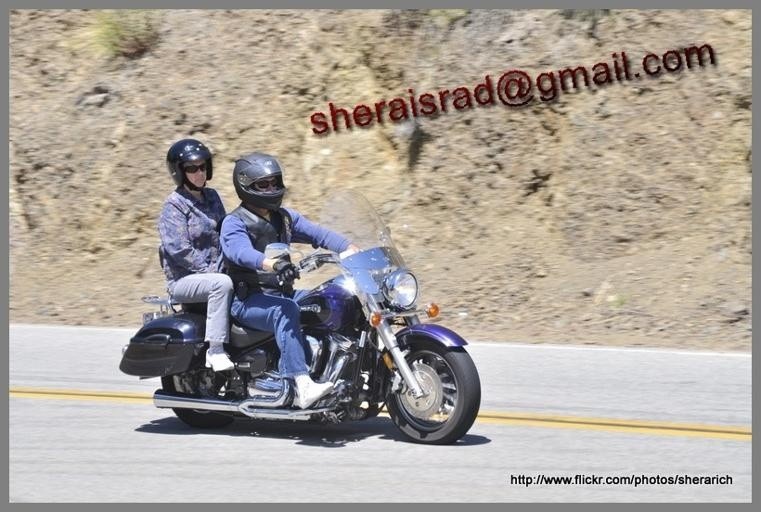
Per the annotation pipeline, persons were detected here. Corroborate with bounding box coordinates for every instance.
[156,137,235,374]
[215,150,364,409]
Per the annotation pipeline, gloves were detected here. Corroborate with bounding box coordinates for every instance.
[272,259,301,285]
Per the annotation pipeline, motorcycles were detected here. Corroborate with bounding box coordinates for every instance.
[119,190,484,445]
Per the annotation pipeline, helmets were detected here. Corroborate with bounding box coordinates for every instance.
[232,151,286,212]
[167,139,213,185]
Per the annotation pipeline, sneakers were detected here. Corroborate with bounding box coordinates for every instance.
[203,347,236,372]
[297,381,334,409]
